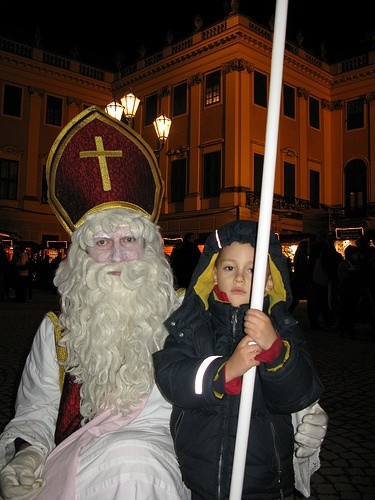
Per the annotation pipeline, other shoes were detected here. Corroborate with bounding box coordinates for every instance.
[335,326,354,336]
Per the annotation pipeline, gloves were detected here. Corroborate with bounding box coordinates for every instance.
[0,446,47,500]
[290,402,327,459]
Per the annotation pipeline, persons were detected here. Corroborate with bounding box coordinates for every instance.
[0,230,375,340]
[152,219,324,500]
[0,208,330,500]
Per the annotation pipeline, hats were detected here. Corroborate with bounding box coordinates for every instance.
[345,245,360,259]
[45,103,166,237]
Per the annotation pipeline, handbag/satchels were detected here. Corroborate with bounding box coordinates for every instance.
[311,247,331,284]
